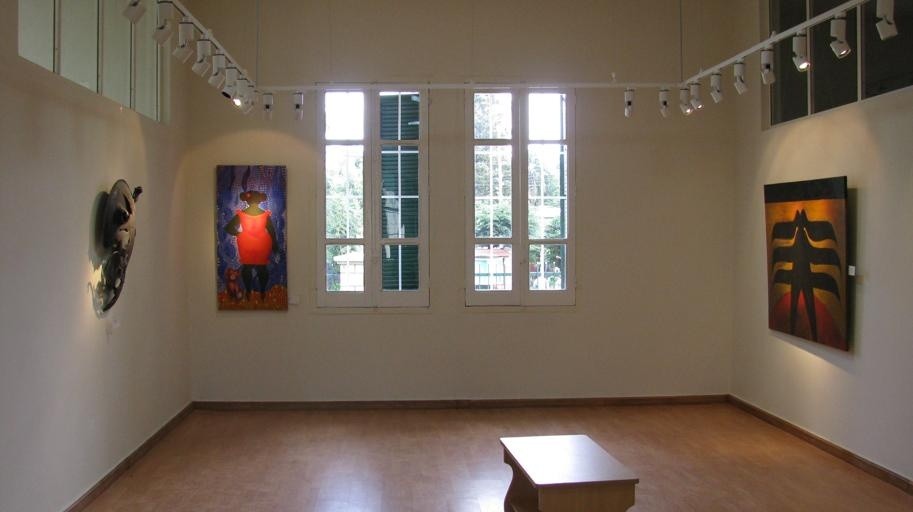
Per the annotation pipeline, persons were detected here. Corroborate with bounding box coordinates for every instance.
[225,190,280,301]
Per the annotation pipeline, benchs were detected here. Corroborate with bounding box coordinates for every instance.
[499,429,641,512]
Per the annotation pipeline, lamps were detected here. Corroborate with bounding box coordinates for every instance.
[622,0,900,117]
[123,0,308,122]
